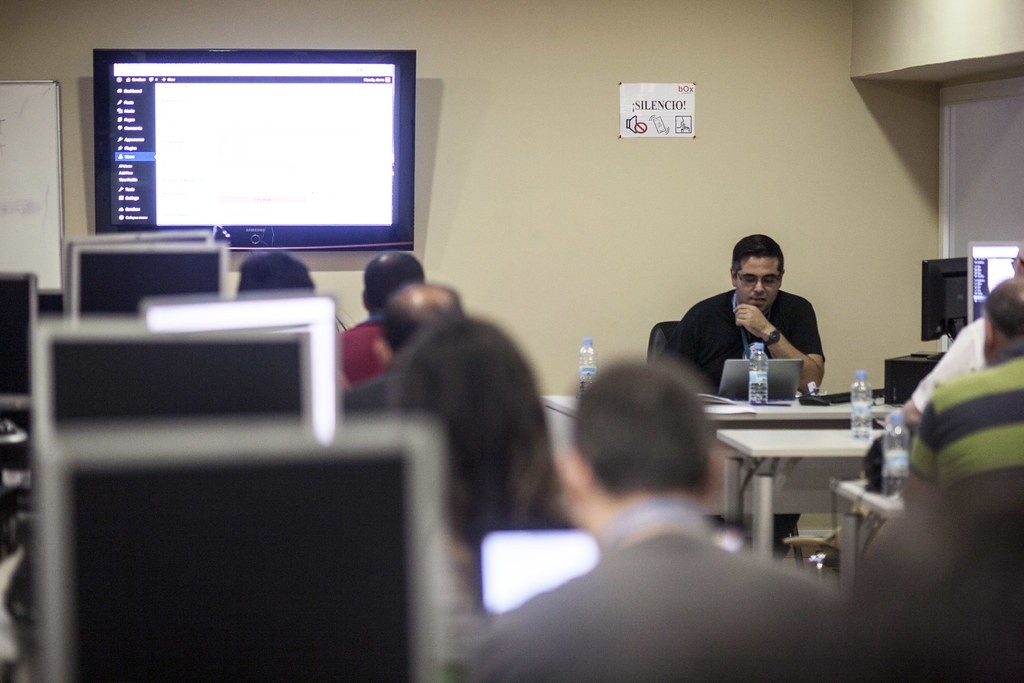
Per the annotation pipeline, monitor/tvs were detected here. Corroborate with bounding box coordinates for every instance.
[1,231,455,683]
[922,256,970,342]
[91,47,416,252]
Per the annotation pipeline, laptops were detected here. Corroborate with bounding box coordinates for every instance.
[478,528,742,616]
[718,359,804,400]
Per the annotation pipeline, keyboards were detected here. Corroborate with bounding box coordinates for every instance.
[820,388,885,404]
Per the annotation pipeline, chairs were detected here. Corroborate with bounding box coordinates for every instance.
[648,322,805,569]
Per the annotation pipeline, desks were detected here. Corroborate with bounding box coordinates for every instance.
[830,477,907,577]
[539,395,883,519]
[717,427,887,563]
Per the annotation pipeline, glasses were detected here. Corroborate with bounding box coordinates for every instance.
[736,272,782,287]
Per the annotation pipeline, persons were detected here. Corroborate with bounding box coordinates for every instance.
[388,318,581,562]
[908,278,1024,486]
[658,234,825,562]
[900,245,1024,427]
[443,362,833,683]
[335,252,425,386]
[343,283,464,415]
[237,254,314,295]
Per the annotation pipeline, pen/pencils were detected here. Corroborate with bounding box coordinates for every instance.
[752,402,792,407]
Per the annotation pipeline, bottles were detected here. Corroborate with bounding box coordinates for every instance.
[748,343,768,404]
[576,337,598,404]
[882,415,910,498]
[850,370,871,440]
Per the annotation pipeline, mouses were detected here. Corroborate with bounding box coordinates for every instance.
[799,396,830,406]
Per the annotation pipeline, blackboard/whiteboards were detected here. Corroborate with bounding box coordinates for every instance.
[0,80,64,294]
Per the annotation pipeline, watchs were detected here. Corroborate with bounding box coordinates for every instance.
[764,329,781,346]
